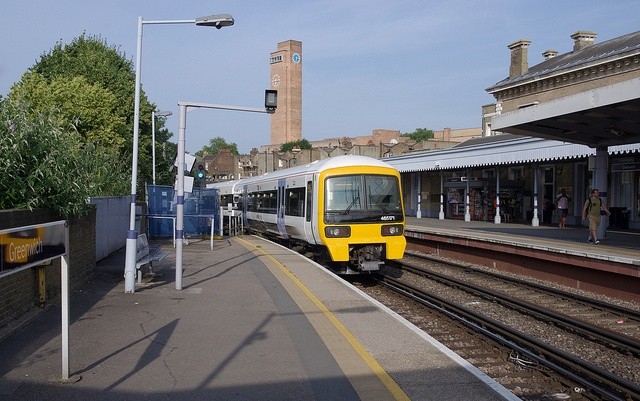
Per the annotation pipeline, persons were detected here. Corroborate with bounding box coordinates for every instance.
[545,198,553,226]
[556,189,570,229]
[582,190,611,244]
[503,200,511,222]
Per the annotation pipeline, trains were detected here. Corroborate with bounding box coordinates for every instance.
[204,154,408,287]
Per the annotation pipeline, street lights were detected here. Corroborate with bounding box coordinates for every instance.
[150,110,173,238]
[175,88,277,291]
[123,13,234,295]
